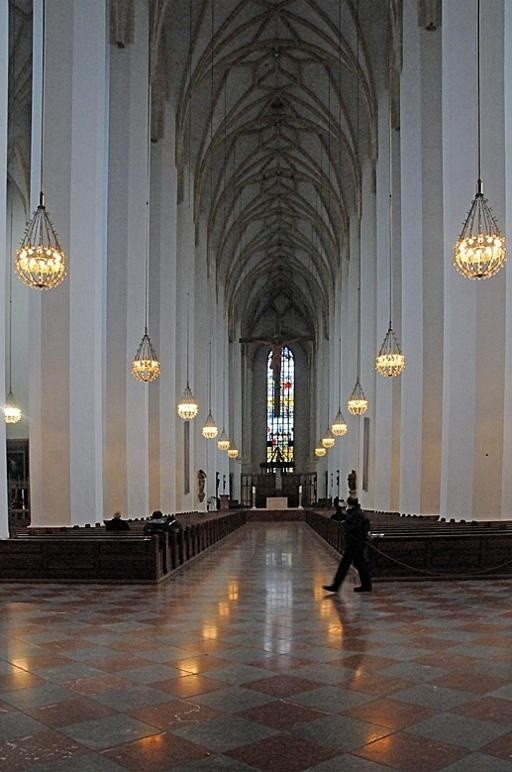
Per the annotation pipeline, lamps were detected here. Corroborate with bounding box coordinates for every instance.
[13,0,67,292]
[178,1,200,420]
[313,2,347,458]
[259,318,296,475]
[1,2,23,426]
[202,0,238,459]
[374,0,404,379]
[129,1,161,383]
[447,0,507,282]
[347,1,368,416]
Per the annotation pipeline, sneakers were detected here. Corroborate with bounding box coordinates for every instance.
[323,586,337,592]
[354,586,372,592]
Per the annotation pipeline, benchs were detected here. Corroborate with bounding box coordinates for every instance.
[303,506,512,584]
[0,509,248,587]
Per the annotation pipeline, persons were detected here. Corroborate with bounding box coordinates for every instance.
[167,514,182,529]
[309,495,347,521]
[143,511,170,530]
[322,495,372,592]
[255,334,305,385]
[105,511,129,531]
